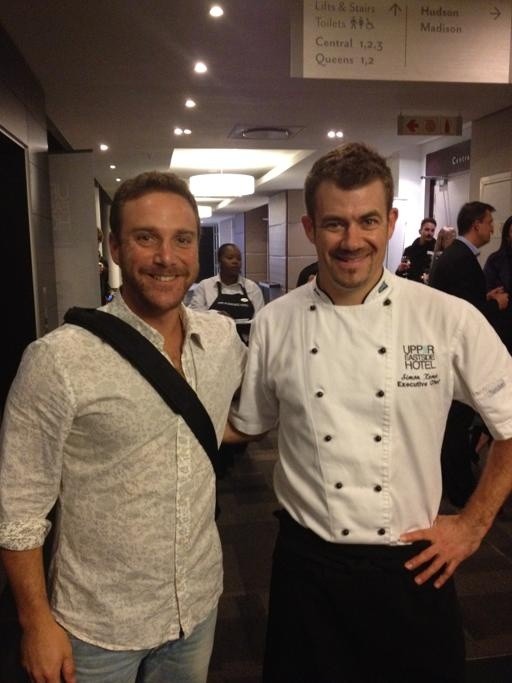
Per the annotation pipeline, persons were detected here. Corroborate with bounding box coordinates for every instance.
[95,225,109,307]
[421,226,456,285]
[427,201,512,509]
[482,213,511,357]
[1,168,254,683]
[186,242,266,346]
[217,139,512,680]
[395,216,439,283]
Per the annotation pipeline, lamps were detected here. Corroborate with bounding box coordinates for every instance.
[189,169,255,197]
[198,206,212,219]
